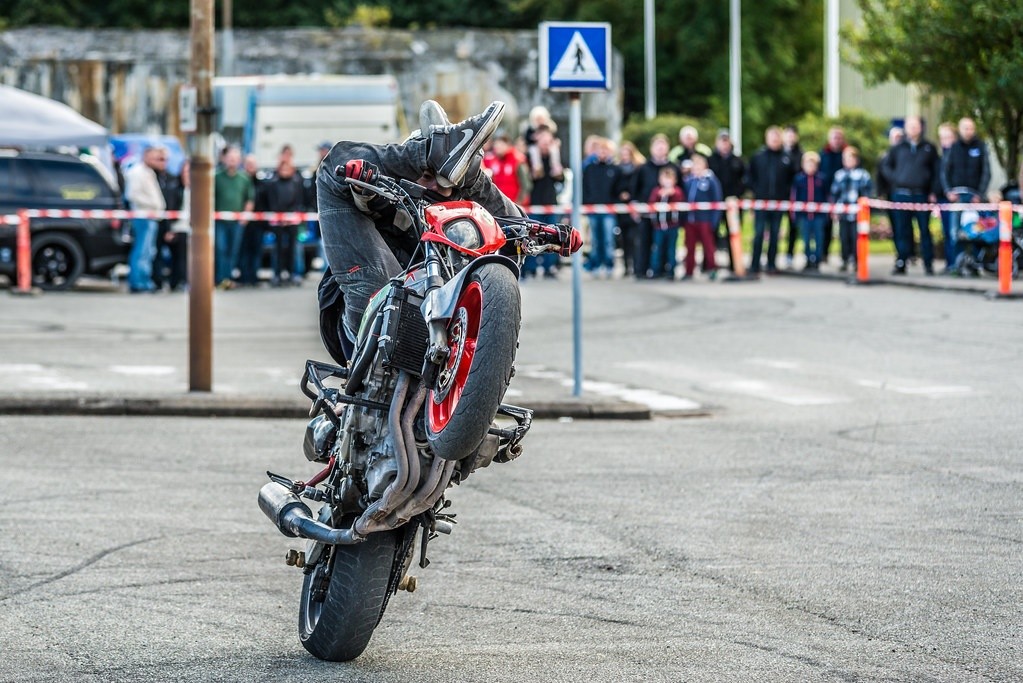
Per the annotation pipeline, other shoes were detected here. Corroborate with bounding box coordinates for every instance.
[750,259,761,273]
[681,272,692,282]
[801,262,820,276]
[767,261,777,274]
[891,259,906,274]
[923,262,935,277]
[785,261,793,270]
[838,265,848,272]
[852,261,858,271]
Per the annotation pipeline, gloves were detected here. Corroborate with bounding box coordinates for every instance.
[536,223,583,257]
[343,159,379,195]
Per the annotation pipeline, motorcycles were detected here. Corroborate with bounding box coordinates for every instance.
[257,164,583,663]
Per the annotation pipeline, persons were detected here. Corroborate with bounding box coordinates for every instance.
[582,123,743,286]
[482,104,568,280]
[874,113,1018,278]
[214,141,333,286]
[743,124,875,276]
[315,99,582,369]
[128,144,193,296]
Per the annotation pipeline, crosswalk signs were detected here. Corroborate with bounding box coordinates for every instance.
[538,22,612,94]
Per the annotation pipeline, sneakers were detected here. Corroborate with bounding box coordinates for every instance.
[419,100,456,189]
[426,101,506,186]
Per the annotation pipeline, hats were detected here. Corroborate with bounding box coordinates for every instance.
[316,141,333,150]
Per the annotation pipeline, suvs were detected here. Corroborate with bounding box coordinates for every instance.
[0,147,131,293]
